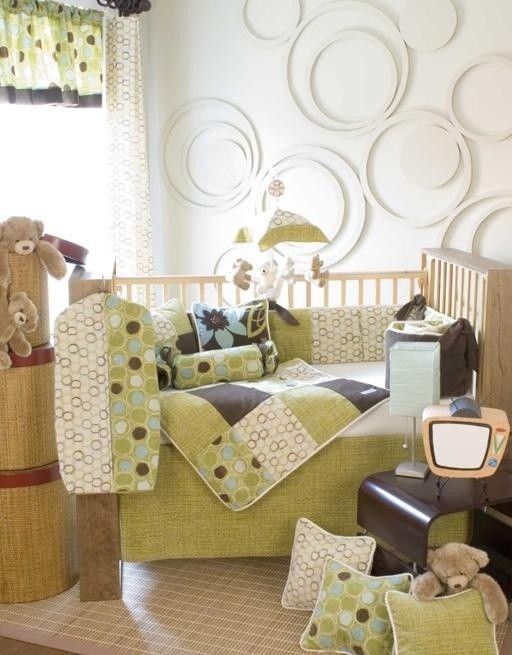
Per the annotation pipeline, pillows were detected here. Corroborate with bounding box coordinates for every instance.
[280,517,500,655]
[147,297,279,391]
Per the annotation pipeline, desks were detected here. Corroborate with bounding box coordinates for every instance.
[354,466,488,583]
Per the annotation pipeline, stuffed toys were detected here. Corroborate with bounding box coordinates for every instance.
[409,542,509,624]
[0,216,68,287]
[230,254,329,296]
[0,277,39,370]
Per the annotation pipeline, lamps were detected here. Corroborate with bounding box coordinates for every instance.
[388,340,440,482]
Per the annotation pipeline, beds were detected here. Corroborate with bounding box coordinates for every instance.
[65,245,511,603]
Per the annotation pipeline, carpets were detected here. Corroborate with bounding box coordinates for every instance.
[0,560,509,654]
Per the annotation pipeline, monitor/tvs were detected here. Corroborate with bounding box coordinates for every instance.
[420,404,511,479]
